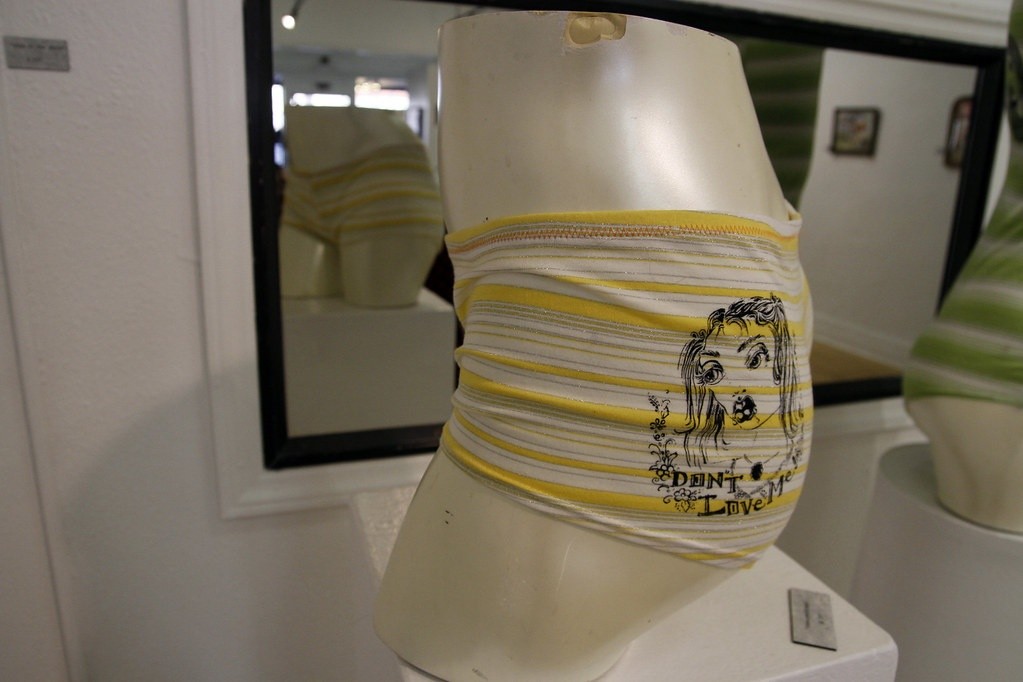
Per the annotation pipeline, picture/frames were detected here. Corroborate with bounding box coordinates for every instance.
[827,107,882,160]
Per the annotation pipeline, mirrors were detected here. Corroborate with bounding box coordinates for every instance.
[241,0,1012,475]
